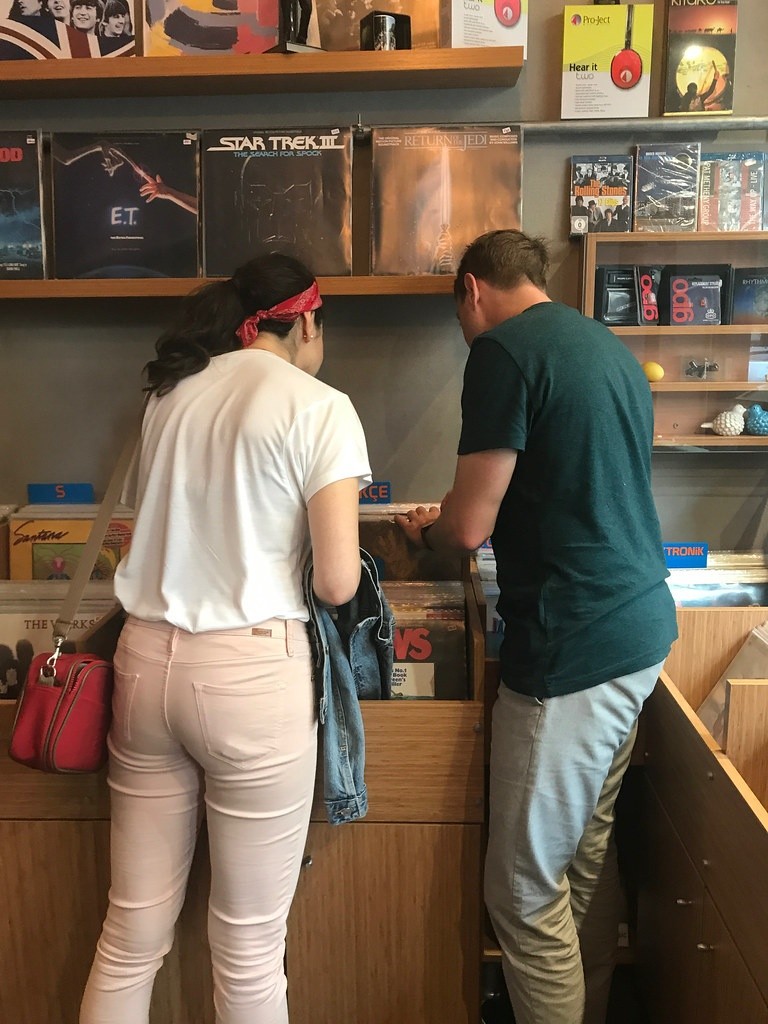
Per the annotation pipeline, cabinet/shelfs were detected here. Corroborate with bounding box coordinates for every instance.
[2,503,765,1024]
[1,46,526,297]
[582,231,768,447]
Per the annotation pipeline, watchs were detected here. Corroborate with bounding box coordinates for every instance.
[419,521,438,552]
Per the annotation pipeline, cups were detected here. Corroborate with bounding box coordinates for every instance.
[374,16,397,51]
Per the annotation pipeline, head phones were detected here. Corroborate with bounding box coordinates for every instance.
[612,4,643,90]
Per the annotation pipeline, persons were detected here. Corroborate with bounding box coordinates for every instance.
[100,0,128,36]
[78,252,375,1024]
[394,228,680,1024]
[46,0,70,24]
[17,0,45,16]
[70,0,102,34]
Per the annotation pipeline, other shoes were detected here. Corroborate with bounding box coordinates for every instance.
[481,995,516,1024]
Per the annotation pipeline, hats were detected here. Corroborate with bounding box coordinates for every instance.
[589,200,596,206]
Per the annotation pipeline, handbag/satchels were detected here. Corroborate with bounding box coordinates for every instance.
[9,653,113,775]
